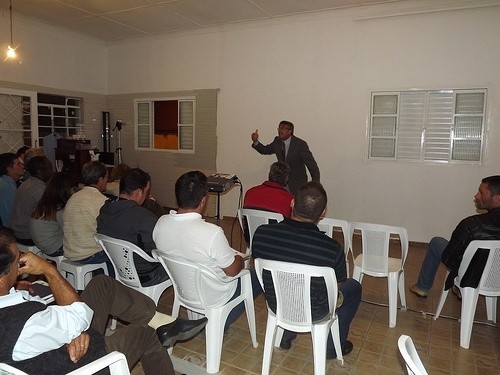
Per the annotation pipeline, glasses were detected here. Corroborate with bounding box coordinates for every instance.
[277,126,292,130]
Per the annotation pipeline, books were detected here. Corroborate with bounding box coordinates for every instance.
[29,283,54,300]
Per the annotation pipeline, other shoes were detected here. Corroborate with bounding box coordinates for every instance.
[452,285,462,301]
[280,332,297,349]
[409,284,428,299]
[327,340,353,359]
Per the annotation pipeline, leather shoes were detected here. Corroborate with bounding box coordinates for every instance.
[156,315,208,347]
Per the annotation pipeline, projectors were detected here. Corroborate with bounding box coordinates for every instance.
[206,172,237,192]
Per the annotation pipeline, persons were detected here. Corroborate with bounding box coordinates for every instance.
[97,168,170,287]
[0,233,209,375]
[251,120,320,196]
[153,170,261,336]
[251,181,362,358]
[63,162,115,280]
[107,163,162,215]
[409,175,500,298]
[0,147,52,246]
[243,163,295,247]
[31,173,76,257]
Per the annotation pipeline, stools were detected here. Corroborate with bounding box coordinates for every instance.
[16,244,109,292]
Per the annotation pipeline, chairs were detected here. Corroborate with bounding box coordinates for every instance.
[433,240,500,350]
[0,351,131,375]
[317,215,350,280]
[150,248,258,374]
[93,232,193,331]
[237,208,284,256]
[348,220,408,328]
[397,333,428,375]
[253,256,345,375]
